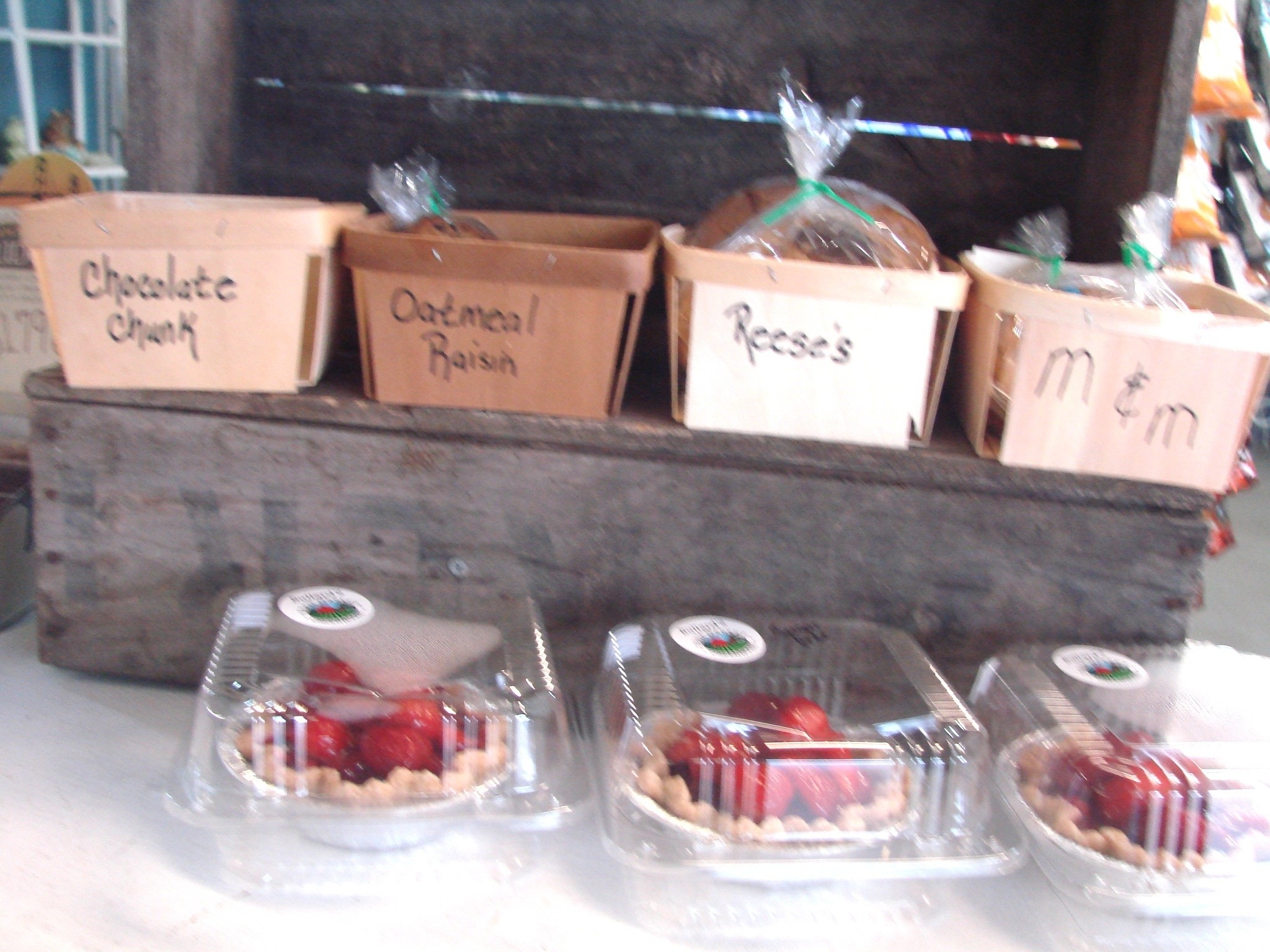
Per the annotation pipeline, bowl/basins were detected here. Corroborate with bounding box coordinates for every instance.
[216,674,519,853]
[609,698,920,859]
[997,722,1269,917]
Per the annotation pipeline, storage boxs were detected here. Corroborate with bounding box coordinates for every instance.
[958,244,1270,492]
[343,211,658,419]
[163,580,602,898]
[18,190,362,395]
[545,613,1031,932]
[655,226,974,450]
[965,638,1270,952]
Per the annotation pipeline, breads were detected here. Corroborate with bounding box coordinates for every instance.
[686,171,943,273]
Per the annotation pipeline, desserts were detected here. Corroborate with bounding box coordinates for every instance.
[232,657,512,808]
[628,687,913,846]
[1010,723,1270,885]
